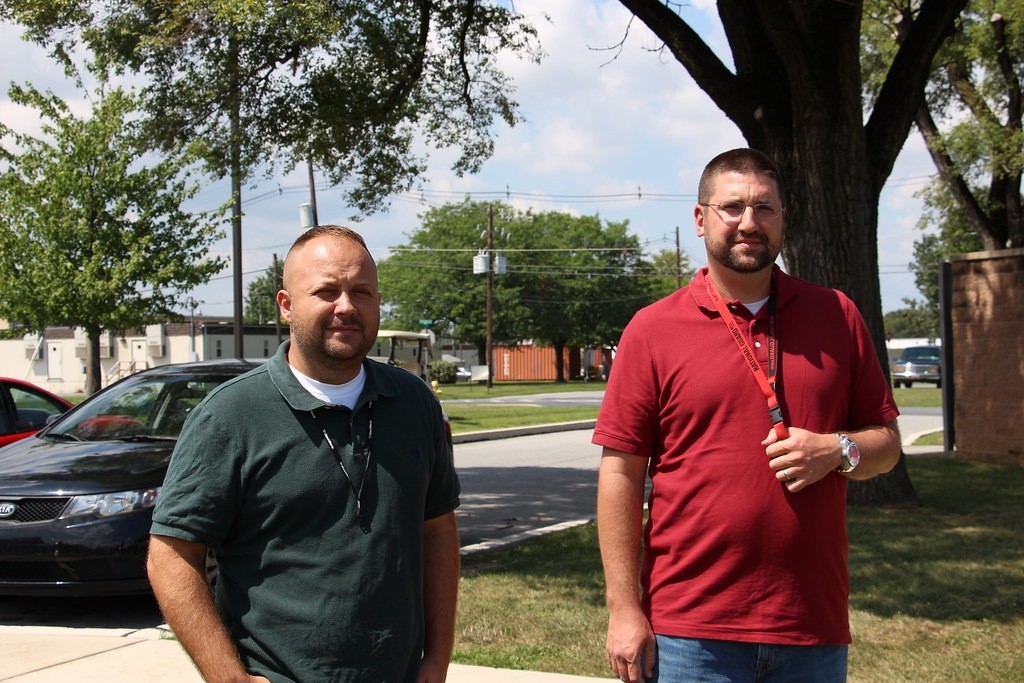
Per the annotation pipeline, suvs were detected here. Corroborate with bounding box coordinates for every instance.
[892,345,941,389]
[367,330,433,385]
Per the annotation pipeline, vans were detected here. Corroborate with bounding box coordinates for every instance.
[0,358,271,600]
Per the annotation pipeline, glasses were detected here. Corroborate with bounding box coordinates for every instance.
[701,200,785,222]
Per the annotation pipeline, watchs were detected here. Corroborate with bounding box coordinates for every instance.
[834,431,860,473]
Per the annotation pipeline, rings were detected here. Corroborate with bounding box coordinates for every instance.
[784,469,790,480]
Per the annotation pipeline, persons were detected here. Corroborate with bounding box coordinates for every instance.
[147,226,461,683]
[591,147,902,683]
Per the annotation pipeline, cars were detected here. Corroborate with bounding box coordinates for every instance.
[456,368,473,383]
[1,376,144,448]
